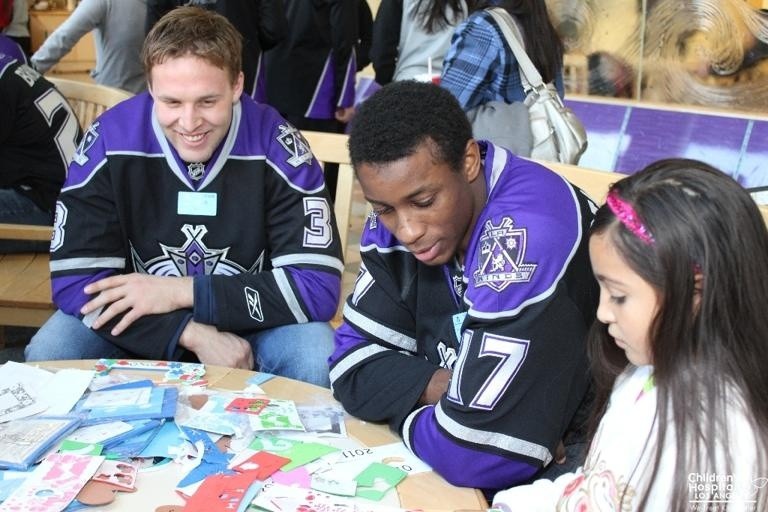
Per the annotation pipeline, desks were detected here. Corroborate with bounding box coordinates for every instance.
[0,358,490,508]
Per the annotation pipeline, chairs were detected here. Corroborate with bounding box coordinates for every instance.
[1,76,768,495]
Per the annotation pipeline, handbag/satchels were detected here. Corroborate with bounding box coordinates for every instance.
[465,6,588,165]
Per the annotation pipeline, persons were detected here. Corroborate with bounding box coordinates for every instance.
[21,6,347,389]
[0,0,563,253]
[326,77,627,503]
[491,157,768,511]
